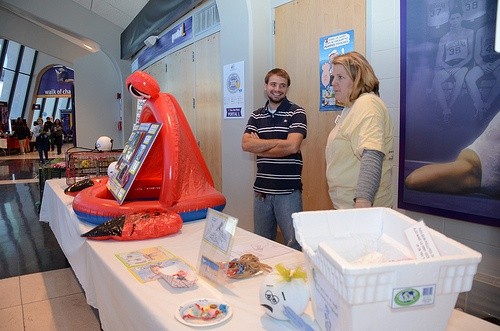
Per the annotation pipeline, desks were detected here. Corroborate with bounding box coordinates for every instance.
[38,175,500,331]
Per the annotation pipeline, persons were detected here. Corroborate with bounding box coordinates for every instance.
[12,116,74,163]
[325,51,395,209]
[241,68,307,251]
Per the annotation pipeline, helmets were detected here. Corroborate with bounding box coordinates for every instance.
[95,136,114,151]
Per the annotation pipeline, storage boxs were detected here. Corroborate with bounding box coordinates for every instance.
[292,207,482,331]
